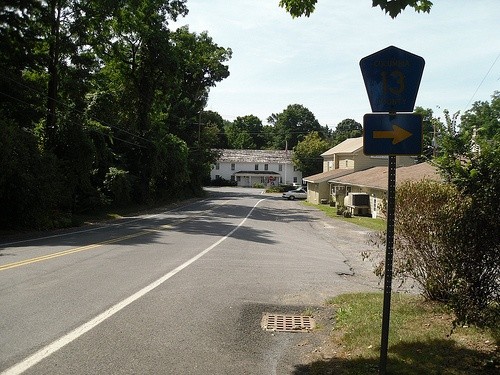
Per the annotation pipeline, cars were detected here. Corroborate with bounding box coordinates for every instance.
[282,188,307,200]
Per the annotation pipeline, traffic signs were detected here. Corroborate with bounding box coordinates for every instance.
[362,113,424,158]
[358,44,426,115]
